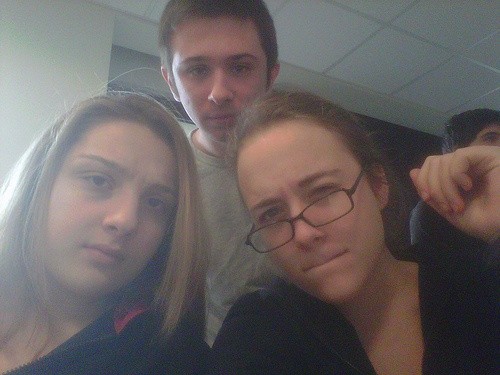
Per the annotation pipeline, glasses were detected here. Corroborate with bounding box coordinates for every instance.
[245,167,365,253]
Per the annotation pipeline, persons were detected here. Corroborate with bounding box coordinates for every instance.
[410,108,500,258]
[0,91,217,375]
[150,0,282,350]
[211,87,500,375]
